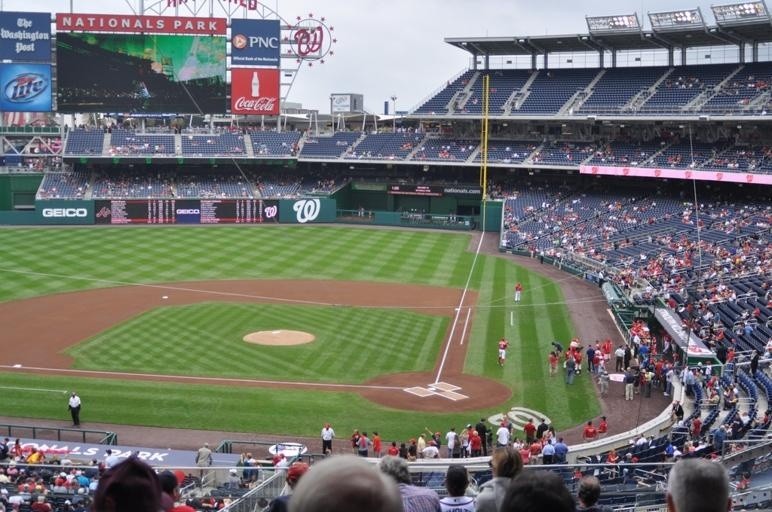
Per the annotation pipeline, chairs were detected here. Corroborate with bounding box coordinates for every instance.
[0,62,772,512]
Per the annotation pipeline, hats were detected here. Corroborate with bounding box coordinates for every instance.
[286,462,309,487]
[95,451,174,512]
[158,469,186,492]
[447,465,467,485]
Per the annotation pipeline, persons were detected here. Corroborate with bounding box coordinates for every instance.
[0,413,772,512]
[498,71,772,415]
[0,76,498,230]
[66,392,82,426]
